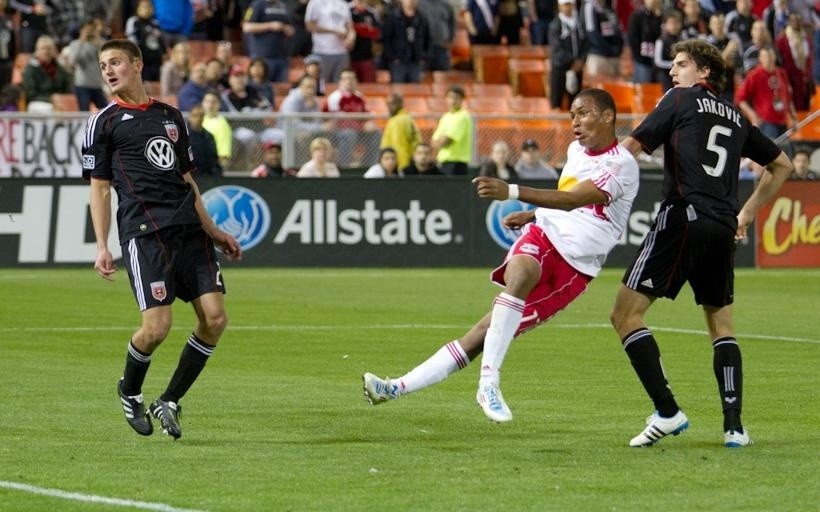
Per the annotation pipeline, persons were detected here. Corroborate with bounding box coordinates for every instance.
[609,41,794,447]
[82,39,243,441]
[458,0,820,181]
[1,0,472,182]
[362,88,640,422]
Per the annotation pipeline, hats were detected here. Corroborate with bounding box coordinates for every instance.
[229,65,244,76]
[263,141,281,151]
[522,139,539,149]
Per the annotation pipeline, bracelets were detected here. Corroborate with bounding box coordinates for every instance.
[507,184,519,200]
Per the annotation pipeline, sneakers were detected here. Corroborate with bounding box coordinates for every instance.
[362,371,392,405]
[116,379,156,434]
[476,387,513,422]
[722,430,750,448]
[629,408,688,452]
[150,398,182,438]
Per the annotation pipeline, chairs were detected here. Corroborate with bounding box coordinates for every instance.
[784,84,820,141]
